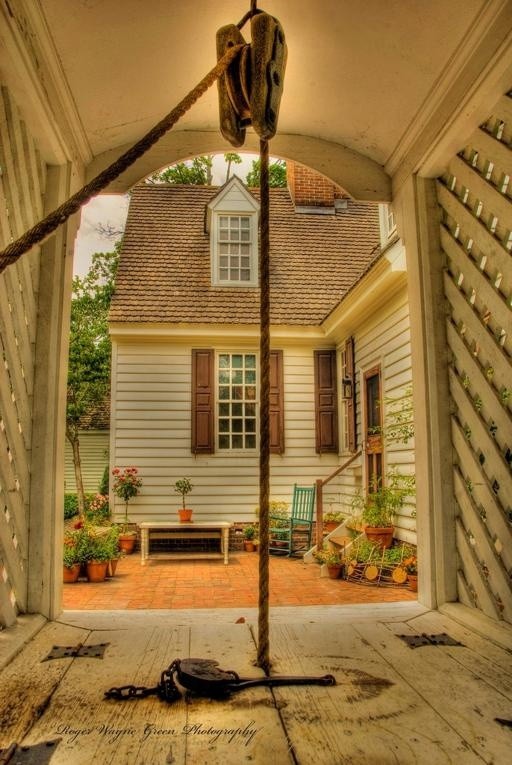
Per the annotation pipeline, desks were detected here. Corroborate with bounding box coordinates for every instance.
[139,521,233,566]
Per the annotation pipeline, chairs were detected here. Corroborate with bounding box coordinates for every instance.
[268,483,315,555]
[352,464,404,548]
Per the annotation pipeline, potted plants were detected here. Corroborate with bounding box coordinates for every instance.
[323,511,344,532]
[174,478,194,522]
[326,548,344,579]
[63,526,118,584]
[243,501,287,554]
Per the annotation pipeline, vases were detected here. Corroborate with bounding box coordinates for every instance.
[119,536,137,553]
[408,575,418,592]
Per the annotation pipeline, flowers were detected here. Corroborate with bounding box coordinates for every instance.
[405,554,418,575]
[112,468,143,533]
